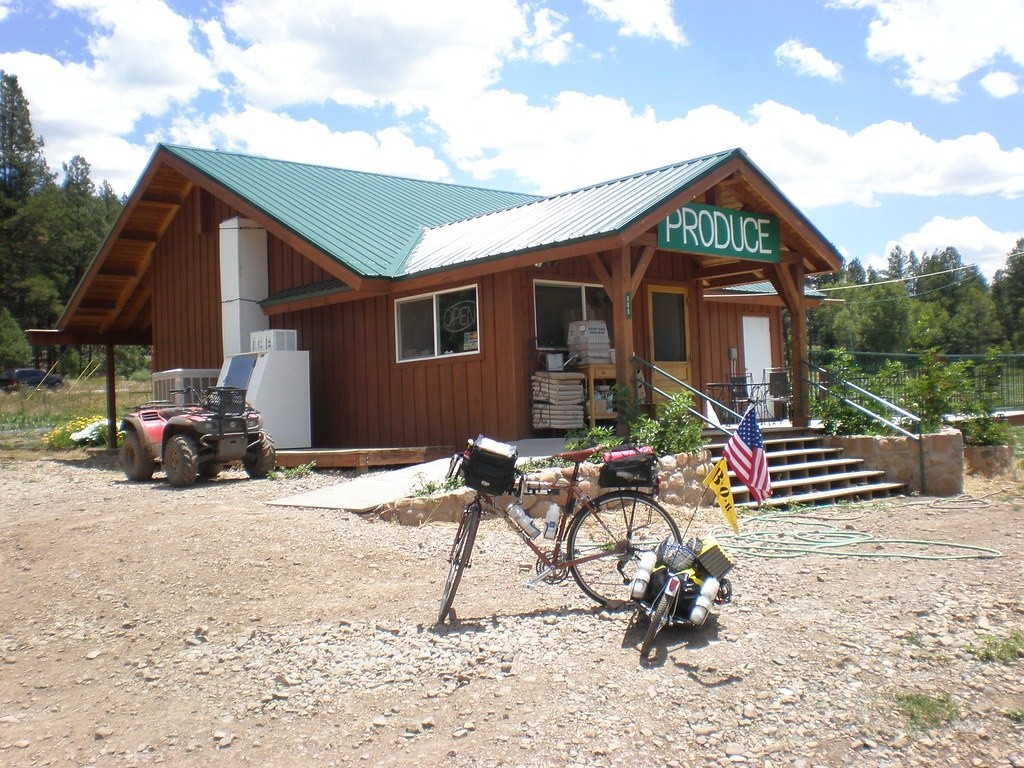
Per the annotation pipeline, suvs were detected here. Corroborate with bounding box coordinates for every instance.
[0,368,64,394]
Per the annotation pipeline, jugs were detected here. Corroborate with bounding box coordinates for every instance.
[538,352,578,371]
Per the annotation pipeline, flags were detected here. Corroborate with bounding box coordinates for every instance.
[722,404,773,506]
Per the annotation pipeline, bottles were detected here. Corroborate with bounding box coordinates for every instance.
[690,577,719,627]
[634,549,656,598]
[544,504,560,540]
[506,499,542,540]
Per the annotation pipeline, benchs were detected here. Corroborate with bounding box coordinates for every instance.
[730,396,792,424]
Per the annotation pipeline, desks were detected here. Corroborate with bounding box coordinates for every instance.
[575,362,619,429]
[707,380,779,427]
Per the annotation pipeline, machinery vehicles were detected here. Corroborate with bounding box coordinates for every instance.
[121,385,277,490]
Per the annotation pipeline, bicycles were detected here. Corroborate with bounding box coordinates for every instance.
[435,448,688,624]
